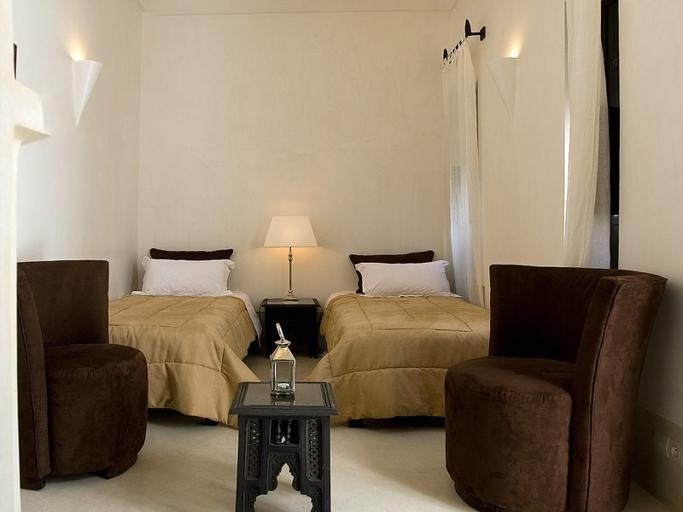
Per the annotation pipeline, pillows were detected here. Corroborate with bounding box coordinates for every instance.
[353,260,462,297]
[130,256,235,295]
[149,247,233,259]
[346,250,436,295]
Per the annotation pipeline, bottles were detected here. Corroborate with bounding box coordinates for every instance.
[269,396,297,405]
[269,321,296,396]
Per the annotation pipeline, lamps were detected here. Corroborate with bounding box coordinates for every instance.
[263,214,318,301]
[485,57,518,126]
[72,60,101,130]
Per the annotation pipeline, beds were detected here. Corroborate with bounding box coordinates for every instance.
[321,289,491,428]
[108,291,262,425]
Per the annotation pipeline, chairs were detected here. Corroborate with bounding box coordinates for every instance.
[444,265,670,510]
[16,259,149,490]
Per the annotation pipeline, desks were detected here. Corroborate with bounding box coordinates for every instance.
[227,379,338,512]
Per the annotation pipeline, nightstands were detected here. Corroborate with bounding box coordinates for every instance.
[259,297,321,359]
